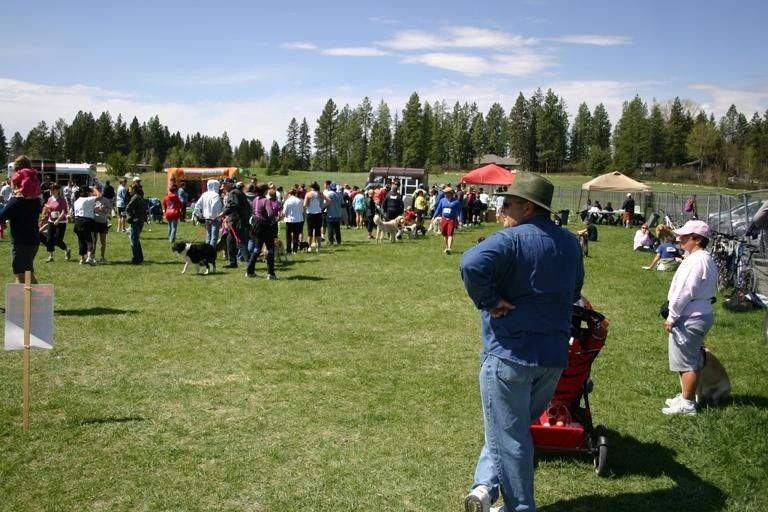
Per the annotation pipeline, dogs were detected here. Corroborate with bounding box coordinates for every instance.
[426,216,442,235]
[681,345,732,403]
[297,239,311,252]
[395,223,427,241]
[172,232,229,276]
[372,213,406,244]
[262,238,289,263]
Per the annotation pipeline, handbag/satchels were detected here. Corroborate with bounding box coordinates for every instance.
[658,301,670,319]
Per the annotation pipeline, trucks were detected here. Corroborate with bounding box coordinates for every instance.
[7,161,105,195]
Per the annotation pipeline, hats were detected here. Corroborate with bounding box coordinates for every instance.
[442,187,454,194]
[256,182,270,191]
[133,177,142,182]
[672,220,712,239]
[221,177,234,183]
[330,182,337,189]
[490,171,562,221]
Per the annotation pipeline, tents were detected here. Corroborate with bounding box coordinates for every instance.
[461,163,513,185]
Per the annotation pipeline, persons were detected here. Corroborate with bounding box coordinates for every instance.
[150,177,405,280]
[455,169,585,512]
[661,219,719,417]
[1,155,148,284]
[404,182,508,254]
[585,182,701,275]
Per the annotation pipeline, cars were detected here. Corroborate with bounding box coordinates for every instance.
[705,200,763,234]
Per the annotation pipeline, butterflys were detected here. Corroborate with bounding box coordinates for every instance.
[655,224,675,244]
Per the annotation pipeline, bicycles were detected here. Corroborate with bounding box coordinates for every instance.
[710,229,760,299]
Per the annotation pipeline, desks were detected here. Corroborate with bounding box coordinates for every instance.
[576,209,645,225]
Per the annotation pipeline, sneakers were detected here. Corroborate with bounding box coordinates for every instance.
[267,272,279,280]
[79,255,107,265]
[64,248,71,260]
[245,272,260,278]
[45,258,54,263]
[222,262,238,268]
[465,484,506,512]
[443,248,452,255]
[662,393,697,418]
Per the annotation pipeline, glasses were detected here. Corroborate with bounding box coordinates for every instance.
[504,200,527,210]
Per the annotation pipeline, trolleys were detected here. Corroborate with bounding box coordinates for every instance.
[526,297,615,476]
